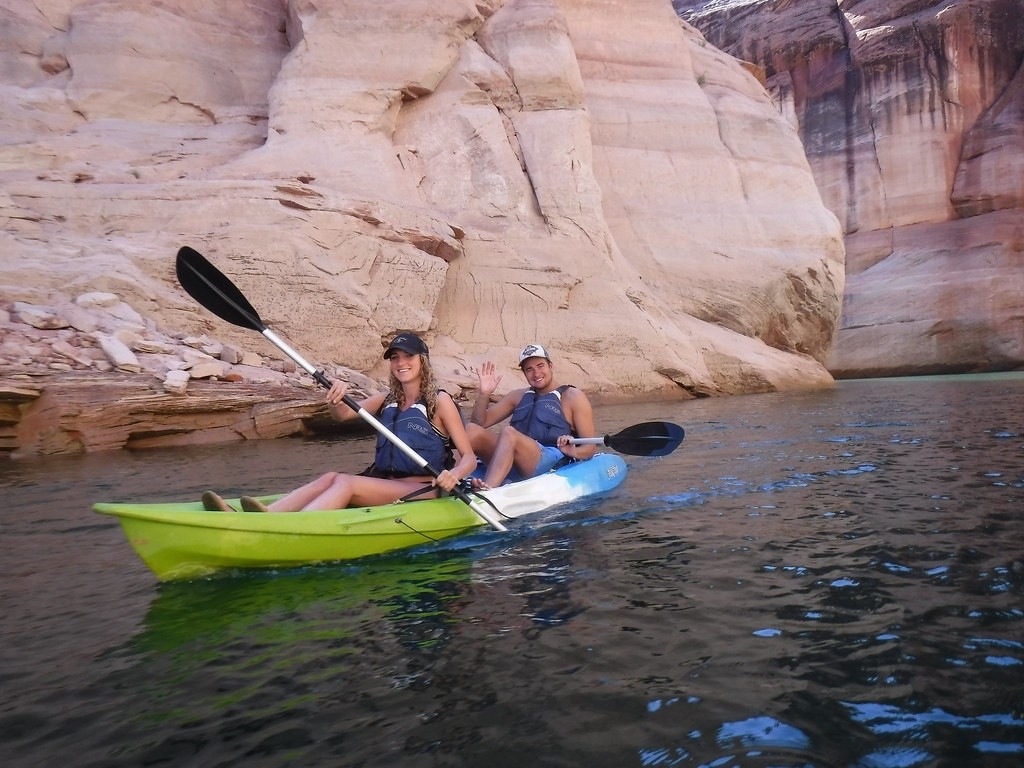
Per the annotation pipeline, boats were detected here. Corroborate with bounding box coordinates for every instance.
[91,443,630,587]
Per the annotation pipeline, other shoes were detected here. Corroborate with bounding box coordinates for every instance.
[200,491,238,512]
[239,496,267,512]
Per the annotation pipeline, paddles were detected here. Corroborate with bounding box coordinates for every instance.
[175,244,509,533]
[450,420,687,457]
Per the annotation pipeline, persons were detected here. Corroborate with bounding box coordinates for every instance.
[202,334,477,512]
[454,343,596,490]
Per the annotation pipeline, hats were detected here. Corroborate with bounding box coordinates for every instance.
[517,344,550,367]
[382,333,429,359]
